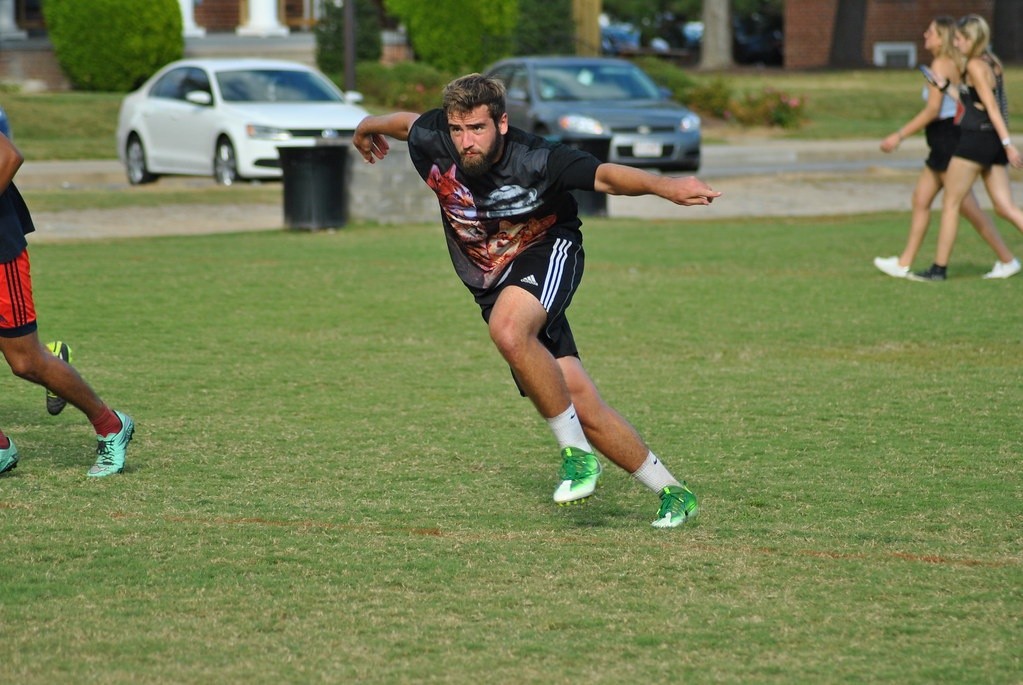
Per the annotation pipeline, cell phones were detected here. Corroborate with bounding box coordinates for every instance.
[919,65,938,86]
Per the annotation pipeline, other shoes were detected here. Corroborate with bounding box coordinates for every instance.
[981,259,1021,279]
[906,265,946,284]
[874,256,910,279]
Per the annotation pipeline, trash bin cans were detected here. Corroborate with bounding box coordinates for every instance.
[276,144,350,231]
[558,134,613,217]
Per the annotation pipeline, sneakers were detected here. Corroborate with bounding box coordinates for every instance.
[44,341,73,416]
[0,437,18,474]
[650,479,700,532]
[552,447,602,506]
[87,409,135,479]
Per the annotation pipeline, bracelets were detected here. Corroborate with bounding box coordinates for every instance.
[939,78,951,92]
[1001,137,1012,146]
[897,130,903,141]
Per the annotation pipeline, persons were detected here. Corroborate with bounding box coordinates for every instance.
[0,110,135,476]
[875,13,1023,285]
[353,73,722,528]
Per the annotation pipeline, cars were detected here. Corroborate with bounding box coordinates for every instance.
[602,19,757,65]
[117,57,369,187]
[480,55,702,174]
